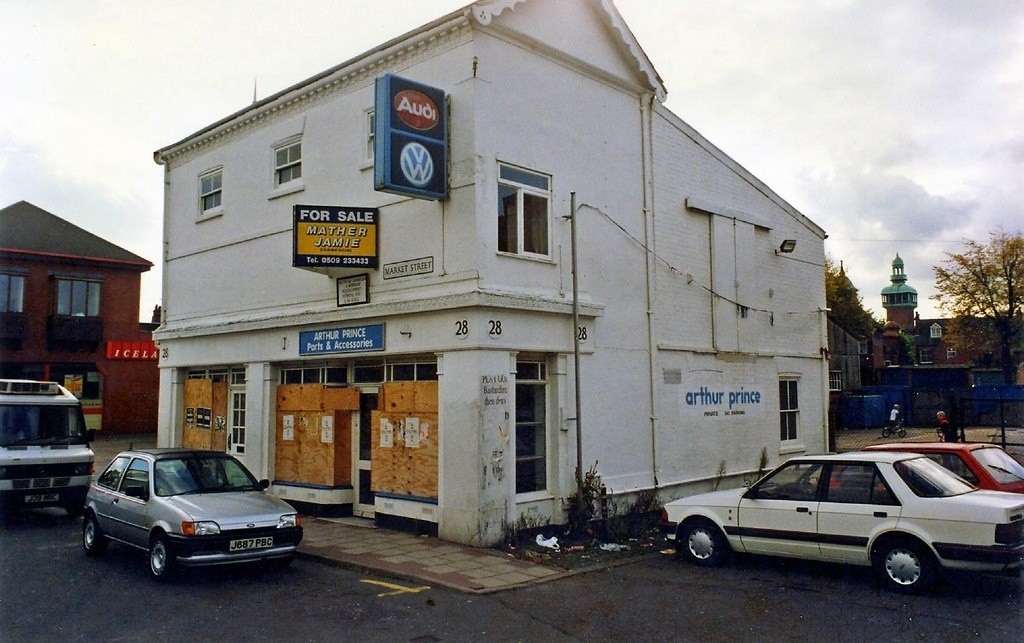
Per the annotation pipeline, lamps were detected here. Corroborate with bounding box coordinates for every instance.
[775,239,797,254]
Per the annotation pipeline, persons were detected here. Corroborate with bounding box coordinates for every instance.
[889,404,902,433]
[937,411,954,442]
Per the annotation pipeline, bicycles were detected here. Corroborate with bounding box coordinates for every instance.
[882,418,906,438]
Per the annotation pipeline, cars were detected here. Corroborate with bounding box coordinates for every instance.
[80,447,303,580]
[657,443,1023,594]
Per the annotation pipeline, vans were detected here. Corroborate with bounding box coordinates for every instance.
[0,378,94,518]
